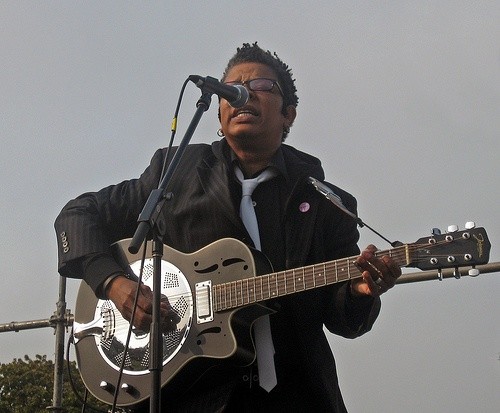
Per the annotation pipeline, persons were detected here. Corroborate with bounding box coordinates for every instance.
[53,41,403,413]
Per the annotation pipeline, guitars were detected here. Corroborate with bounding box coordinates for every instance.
[73,222,492,406]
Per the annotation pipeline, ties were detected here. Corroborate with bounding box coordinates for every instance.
[233,166,279,391]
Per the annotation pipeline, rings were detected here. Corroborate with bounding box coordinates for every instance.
[375,277,382,284]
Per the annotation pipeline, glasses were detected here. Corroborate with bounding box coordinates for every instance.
[223,78,285,97]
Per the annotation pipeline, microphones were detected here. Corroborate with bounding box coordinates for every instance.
[189,75,249,109]
